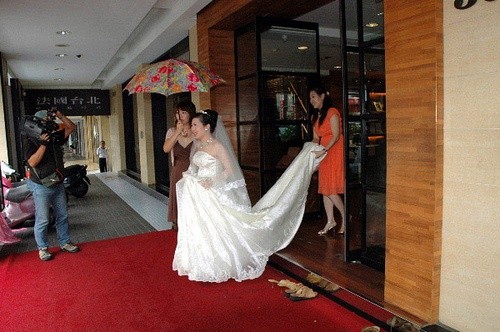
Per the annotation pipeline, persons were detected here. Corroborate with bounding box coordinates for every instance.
[25,107,79,261]
[172,110,328,283]
[163,99,196,230]
[310,85,344,235]
[96,141,109,173]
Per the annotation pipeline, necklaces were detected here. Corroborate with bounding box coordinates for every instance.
[198,137,216,150]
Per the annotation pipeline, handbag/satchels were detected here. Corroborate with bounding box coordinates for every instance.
[39,172,60,188]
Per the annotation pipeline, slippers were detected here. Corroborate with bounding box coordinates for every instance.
[319,279,341,292]
[284,283,318,301]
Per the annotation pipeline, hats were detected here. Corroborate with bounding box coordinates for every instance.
[33,110,48,120]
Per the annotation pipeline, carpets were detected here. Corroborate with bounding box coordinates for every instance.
[0,229,388,332]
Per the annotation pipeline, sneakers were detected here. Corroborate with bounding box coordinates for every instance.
[38,246,51,261]
[60,240,79,252]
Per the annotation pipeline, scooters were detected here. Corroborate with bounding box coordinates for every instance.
[0,160,91,230]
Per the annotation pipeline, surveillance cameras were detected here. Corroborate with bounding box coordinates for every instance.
[76,54,81,57]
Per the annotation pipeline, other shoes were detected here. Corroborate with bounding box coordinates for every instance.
[386,316,420,332]
[362,325,381,332]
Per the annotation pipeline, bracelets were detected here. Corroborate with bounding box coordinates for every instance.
[323,149,327,152]
[59,114,63,119]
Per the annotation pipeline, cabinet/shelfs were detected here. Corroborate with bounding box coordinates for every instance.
[329,70,387,157]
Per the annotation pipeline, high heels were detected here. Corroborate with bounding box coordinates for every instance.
[317,217,338,234]
[338,214,352,233]
[267,278,296,293]
[307,272,324,284]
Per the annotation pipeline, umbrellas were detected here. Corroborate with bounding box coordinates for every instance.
[122,50,228,120]
